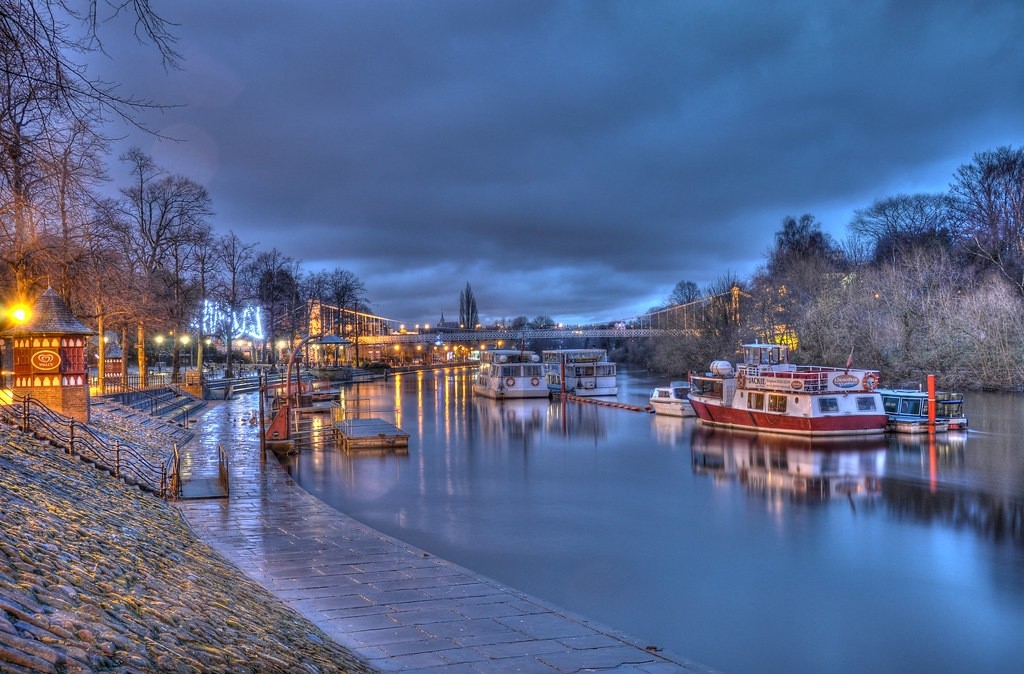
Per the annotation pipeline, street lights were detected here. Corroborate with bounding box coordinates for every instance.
[206,340,211,366]
[182,335,189,371]
[154,336,163,374]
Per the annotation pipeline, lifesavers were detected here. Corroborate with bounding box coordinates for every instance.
[531,377,540,386]
[506,378,516,387]
[862,373,879,391]
[737,372,745,388]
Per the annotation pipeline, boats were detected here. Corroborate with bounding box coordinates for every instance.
[542,350,618,397]
[473,350,551,398]
[649,380,698,417]
[689,422,890,502]
[687,344,889,437]
[873,383,968,435]
